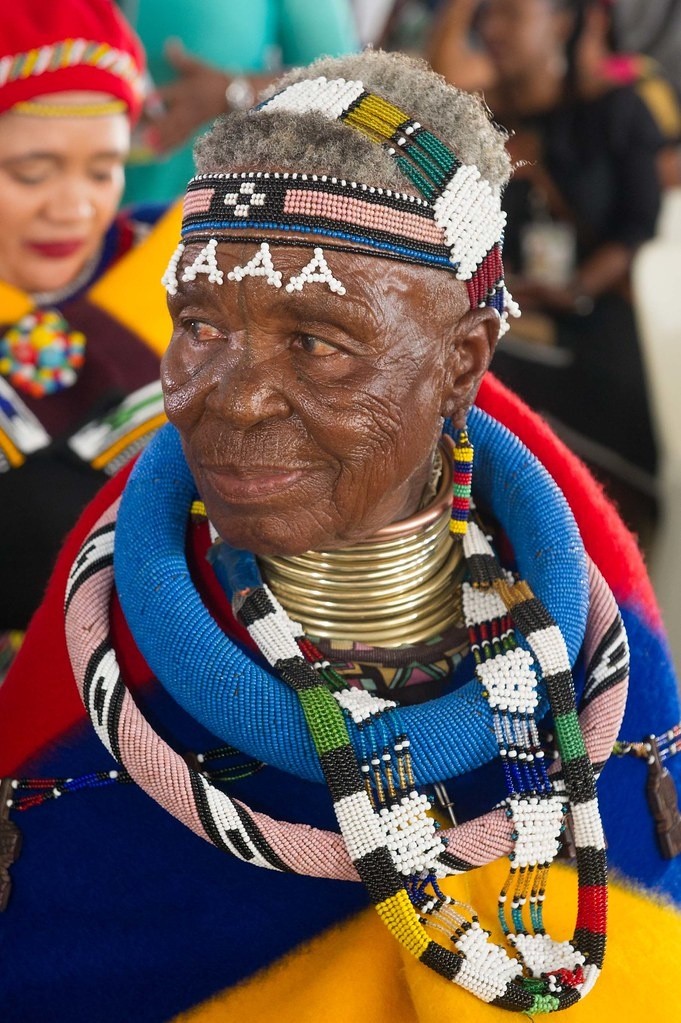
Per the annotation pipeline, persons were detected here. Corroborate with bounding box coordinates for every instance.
[0,0,680,682]
[0,45,681,1023]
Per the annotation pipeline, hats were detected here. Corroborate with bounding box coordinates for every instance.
[0,0,146,131]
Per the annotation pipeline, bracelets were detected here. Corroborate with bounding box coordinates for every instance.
[224,73,256,111]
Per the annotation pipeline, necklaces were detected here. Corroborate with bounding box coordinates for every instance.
[61,447,630,1015]
